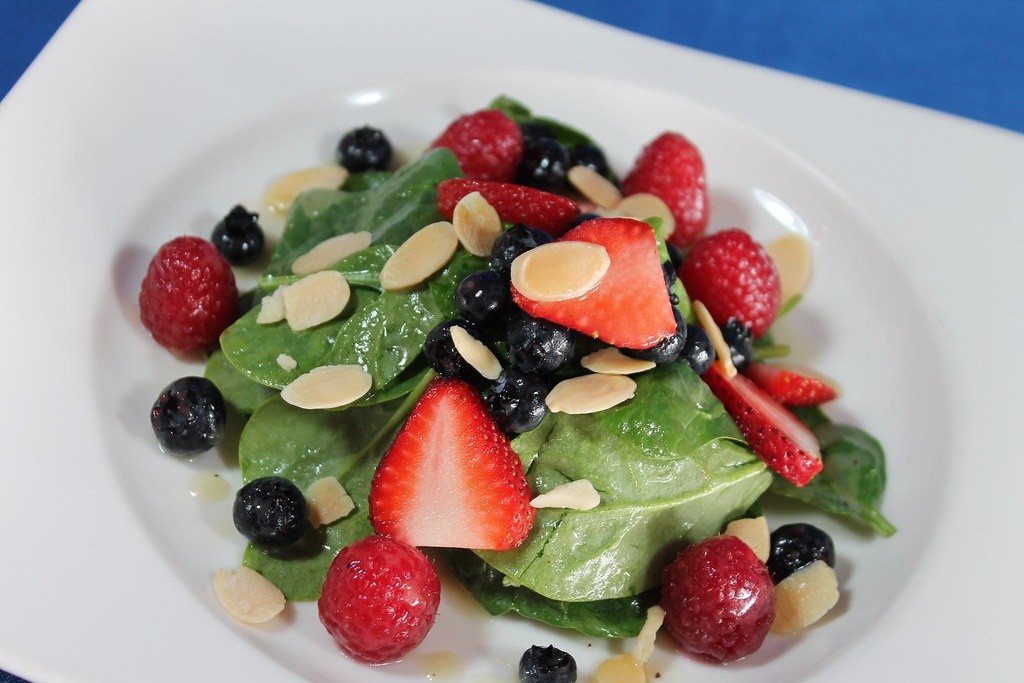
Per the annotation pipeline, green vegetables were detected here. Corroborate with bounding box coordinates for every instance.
[203,101,890,638]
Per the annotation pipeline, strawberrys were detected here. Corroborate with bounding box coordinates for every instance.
[425,111,520,182]
[680,231,781,337]
[748,364,835,407]
[661,534,775,662]
[438,181,580,233]
[623,132,710,242]
[368,382,533,550]
[317,540,440,663]
[512,217,675,350]
[708,368,822,484]
[138,235,236,351]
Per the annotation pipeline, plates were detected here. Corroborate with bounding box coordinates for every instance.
[0,0,1024,683]
